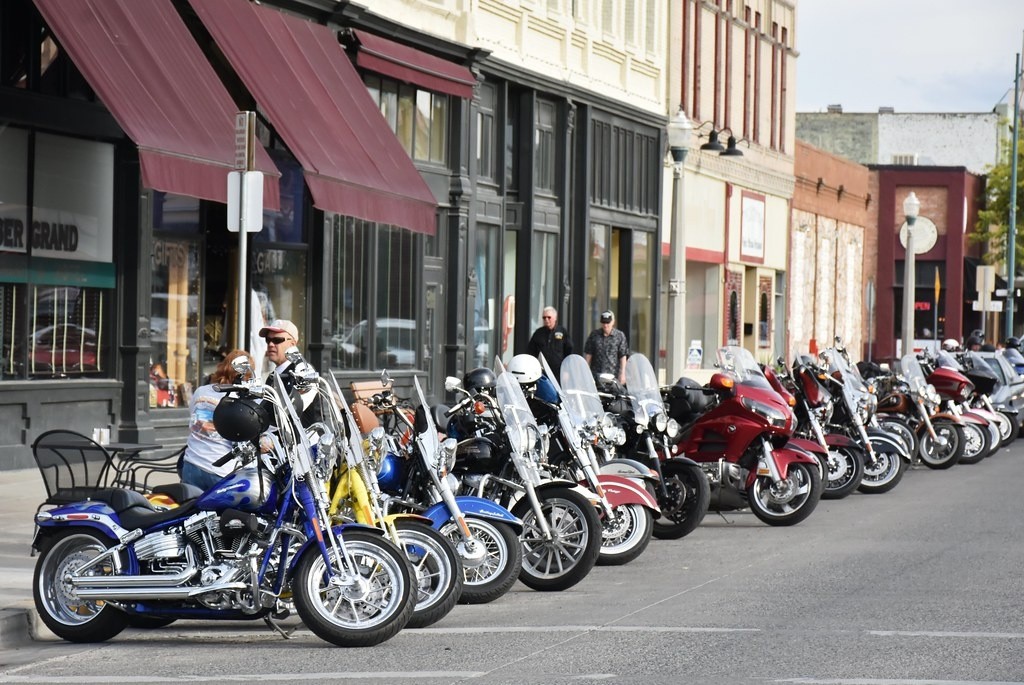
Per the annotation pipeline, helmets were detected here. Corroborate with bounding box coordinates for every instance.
[463,368,496,390]
[1006,337,1021,347]
[506,354,542,383]
[971,330,984,340]
[966,336,981,349]
[212,396,271,443]
[943,338,959,350]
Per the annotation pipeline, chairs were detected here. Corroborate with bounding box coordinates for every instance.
[116,445,188,495]
[30,430,136,557]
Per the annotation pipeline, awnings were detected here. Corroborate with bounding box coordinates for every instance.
[34,0,439,236]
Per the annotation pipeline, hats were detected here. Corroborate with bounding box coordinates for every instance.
[258,319,298,342]
[600,310,615,322]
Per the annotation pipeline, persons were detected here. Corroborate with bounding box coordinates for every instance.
[182,350,273,491]
[259,320,325,449]
[583,310,629,385]
[529,306,574,388]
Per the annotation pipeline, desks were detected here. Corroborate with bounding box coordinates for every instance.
[30,441,163,488]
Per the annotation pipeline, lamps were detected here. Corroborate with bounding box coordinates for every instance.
[663,107,751,167]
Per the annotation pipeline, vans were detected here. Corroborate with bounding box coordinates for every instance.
[340,318,416,366]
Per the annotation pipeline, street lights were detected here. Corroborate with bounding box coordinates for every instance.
[900,191,921,361]
[664,106,695,389]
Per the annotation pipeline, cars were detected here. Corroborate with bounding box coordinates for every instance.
[148,292,198,337]
[149,327,199,369]
[8,322,97,370]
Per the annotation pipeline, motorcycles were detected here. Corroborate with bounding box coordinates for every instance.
[659,345,822,527]
[421,376,603,593]
[596,353,711,540]
[29,346,418,649]
[335,369,524,605]
[111,368,464,629]
[502,352,663,566]
[756,335,1024,500]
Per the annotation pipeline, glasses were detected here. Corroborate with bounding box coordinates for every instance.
[543,316,552,319]
[266,336,293,344]
